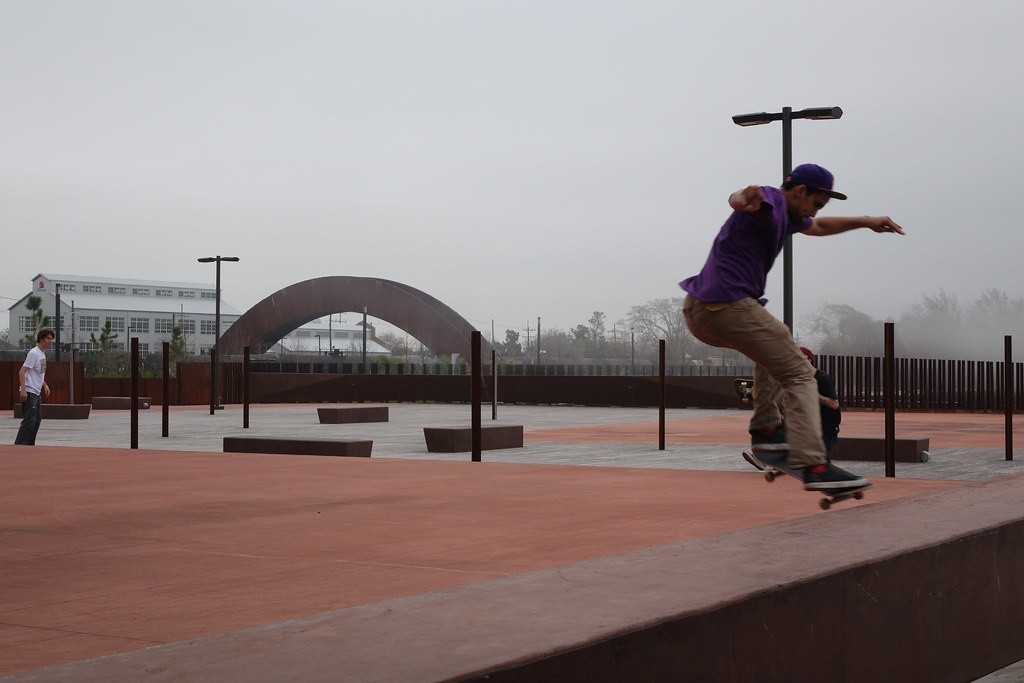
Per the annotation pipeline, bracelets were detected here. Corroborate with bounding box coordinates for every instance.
[44,385,48,387]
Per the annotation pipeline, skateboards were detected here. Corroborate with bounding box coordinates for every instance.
[752,445,875,511]
[733,377,786,406]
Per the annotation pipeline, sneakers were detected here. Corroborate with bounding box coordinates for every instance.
[750,426,792,451]
[802,462,871,491]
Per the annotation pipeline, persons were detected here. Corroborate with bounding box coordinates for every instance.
[742,347,841,470]
[15,329,55,445]
[678,163,905,491]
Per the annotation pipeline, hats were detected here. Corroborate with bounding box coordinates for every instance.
[785,163,847,200]
[799,347,816,366]
